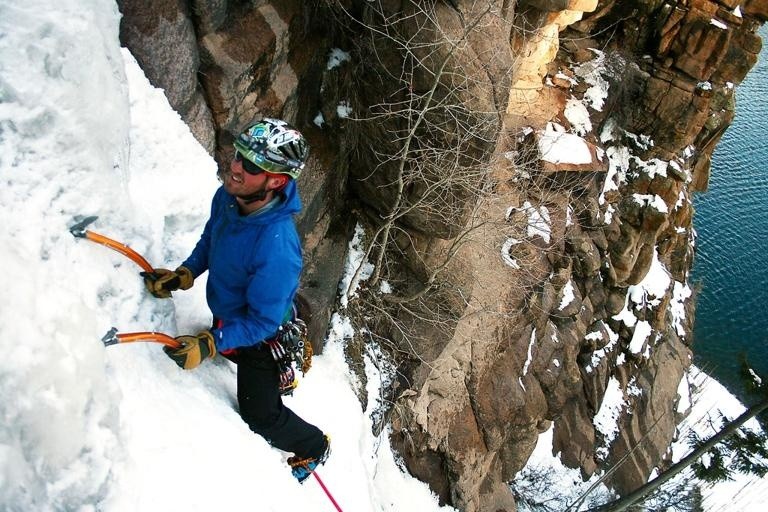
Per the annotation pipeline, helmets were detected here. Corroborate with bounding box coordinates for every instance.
[231,117,311,180]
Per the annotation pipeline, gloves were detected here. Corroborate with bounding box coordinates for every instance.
[162,330,216,370]
[143,266,193,298]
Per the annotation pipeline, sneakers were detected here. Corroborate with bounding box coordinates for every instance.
[286,434,330,485]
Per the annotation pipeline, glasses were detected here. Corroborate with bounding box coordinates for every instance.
[234,149,264,175]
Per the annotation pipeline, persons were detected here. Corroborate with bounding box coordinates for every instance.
[144,118,333,485]
